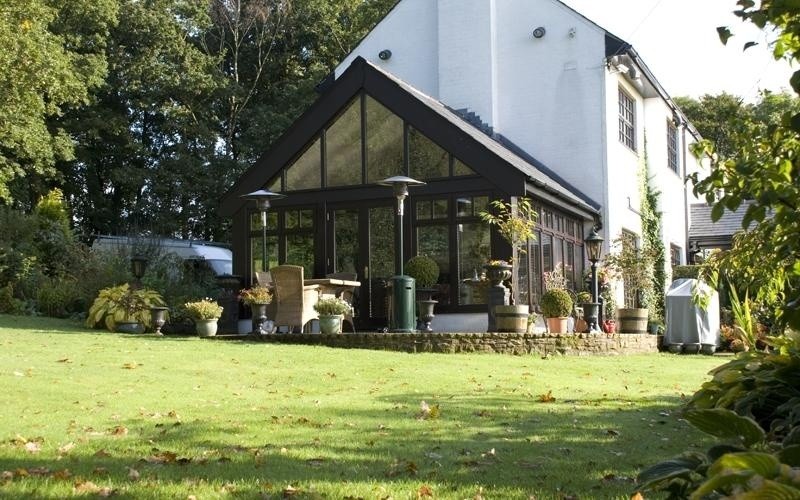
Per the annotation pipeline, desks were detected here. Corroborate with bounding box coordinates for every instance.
[303,278,362,332]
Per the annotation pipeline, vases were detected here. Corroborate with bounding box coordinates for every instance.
[667,342,717,355]
[582,302,601,333]
[417,299,439,331]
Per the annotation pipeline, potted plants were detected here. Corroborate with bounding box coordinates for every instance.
[184,298,224,337]
[238,285,274,334]
[85,281,172,334]
[602,232,655,333]
[478,192,540,333]
[540,290,574,334]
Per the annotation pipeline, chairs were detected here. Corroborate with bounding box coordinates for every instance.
[255,264,358,333]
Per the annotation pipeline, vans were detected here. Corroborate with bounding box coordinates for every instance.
[91,238,233,281]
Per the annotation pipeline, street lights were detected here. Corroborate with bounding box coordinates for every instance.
[585,225,605,334]
